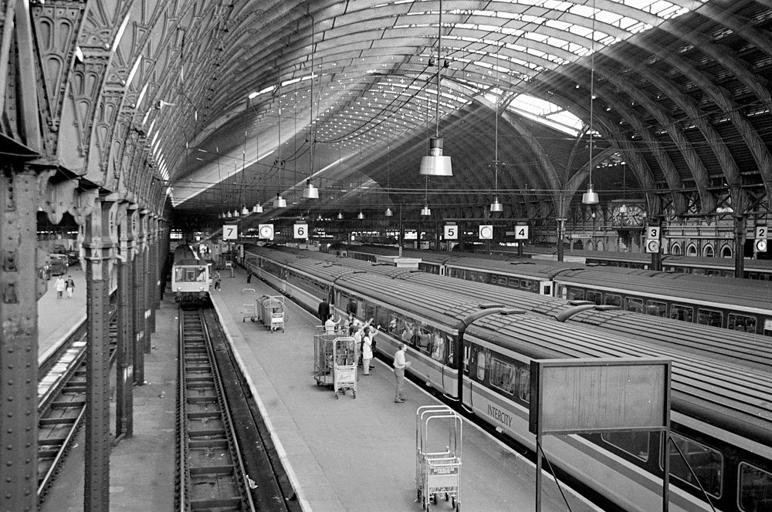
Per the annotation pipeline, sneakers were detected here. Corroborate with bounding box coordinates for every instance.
[369,365,375,370]
[395,398,406,403]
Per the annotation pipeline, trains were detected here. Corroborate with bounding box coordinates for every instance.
[171,242,214,310]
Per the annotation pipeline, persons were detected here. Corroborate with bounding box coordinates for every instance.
[324,314,381,374]
[54,274,75,299]
[387,314,445,363]
[448,351,454,366]
[317,297,330,331]
[346,298,357,318]
[214,271,221,291]
[392,344,413,404]
[247,265,253,283]
